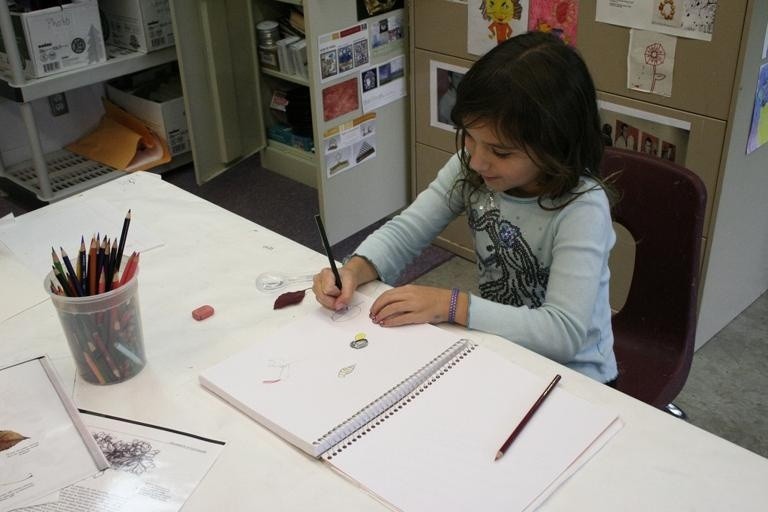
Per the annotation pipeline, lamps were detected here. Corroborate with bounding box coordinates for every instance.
[588,145,708,421]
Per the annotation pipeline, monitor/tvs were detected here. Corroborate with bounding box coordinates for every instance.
[199,291,621,511]
[271,7,308,78]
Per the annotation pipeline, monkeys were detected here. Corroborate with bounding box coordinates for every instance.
[193,306,213,321]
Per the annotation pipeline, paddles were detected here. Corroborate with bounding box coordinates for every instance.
[0,157,457,289]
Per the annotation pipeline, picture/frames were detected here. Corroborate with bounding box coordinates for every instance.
[0,170,768,510]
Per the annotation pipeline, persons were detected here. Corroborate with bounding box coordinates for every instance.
[600,122,673,161]
[439,71,467,125]
[313,32,620,388]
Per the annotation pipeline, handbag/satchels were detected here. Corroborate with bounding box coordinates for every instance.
[448,288,459,322]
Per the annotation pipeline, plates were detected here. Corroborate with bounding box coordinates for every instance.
[409,0,767,353]
[166,0,409,250]
[0,0,193,205]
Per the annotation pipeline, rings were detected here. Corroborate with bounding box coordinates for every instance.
[50,208,145,385]
[315,214,350,310]
[494,374,562,461]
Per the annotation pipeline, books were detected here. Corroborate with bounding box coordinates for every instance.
[97,1,175,55]
[105,60,191,157]
[0,0,108,79]
